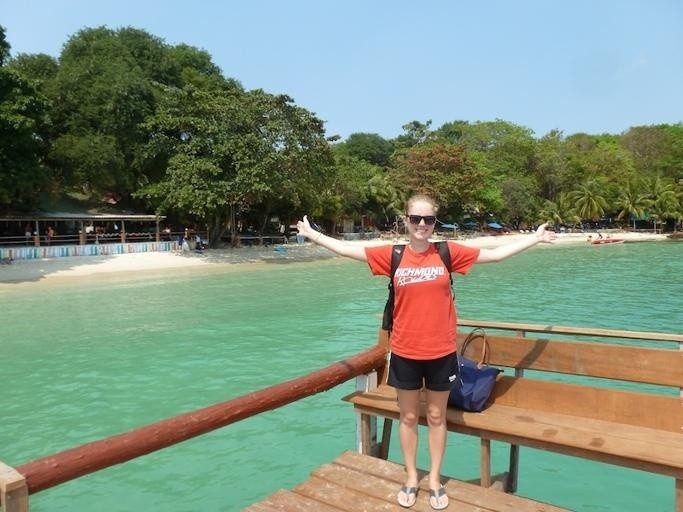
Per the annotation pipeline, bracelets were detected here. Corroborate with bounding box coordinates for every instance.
[313,232,324,243]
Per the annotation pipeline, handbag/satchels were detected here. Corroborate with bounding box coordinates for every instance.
[446,327,504,413]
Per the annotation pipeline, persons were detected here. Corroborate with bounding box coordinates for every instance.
[45,226,55,246]
[586,232,604,242]
[24,223,33,246]
[181,228,205,251]
[295,193,557,510]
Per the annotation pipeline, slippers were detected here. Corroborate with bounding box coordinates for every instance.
[398,484,420,509]
[428,483,448,510]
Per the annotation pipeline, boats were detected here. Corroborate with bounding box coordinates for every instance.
[588,236,629,246]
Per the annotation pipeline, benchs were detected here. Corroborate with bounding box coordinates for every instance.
[340,325,683,512]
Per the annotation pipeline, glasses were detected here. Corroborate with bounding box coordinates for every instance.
[405,215,436,225]
